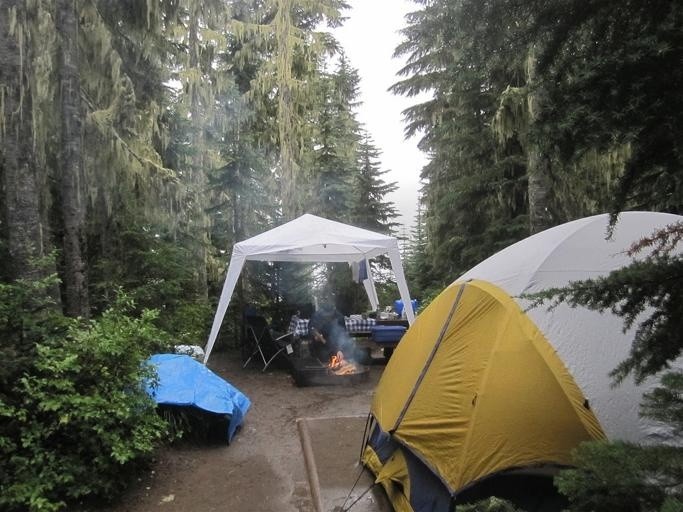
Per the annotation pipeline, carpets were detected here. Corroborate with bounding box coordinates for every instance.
[297,414,393,511]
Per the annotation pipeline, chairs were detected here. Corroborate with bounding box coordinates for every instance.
[240,315,294,373]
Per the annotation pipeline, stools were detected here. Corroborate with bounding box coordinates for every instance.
[371,324,407,358]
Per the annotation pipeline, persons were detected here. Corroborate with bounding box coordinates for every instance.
[307,303,345,363]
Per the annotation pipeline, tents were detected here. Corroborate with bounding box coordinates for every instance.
[356,211,683,511]
